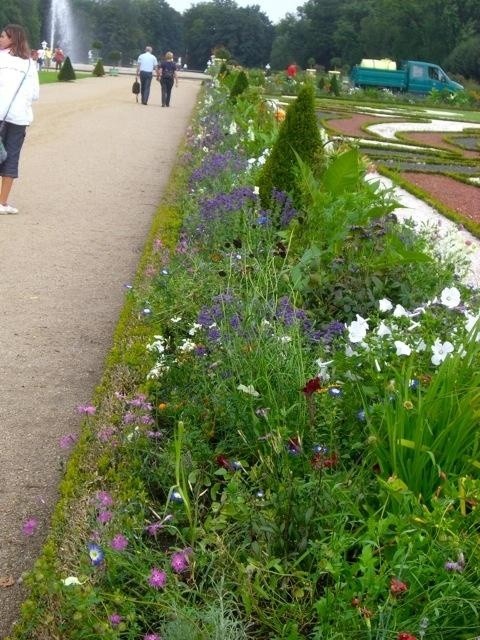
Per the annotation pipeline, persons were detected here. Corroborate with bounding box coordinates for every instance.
[36,44,65,71]
[135,45,161,107]
[158,51,179,108]
[0,22,41,215]
[286,60,298,81]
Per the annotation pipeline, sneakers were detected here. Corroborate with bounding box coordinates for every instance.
[0,203,18,215]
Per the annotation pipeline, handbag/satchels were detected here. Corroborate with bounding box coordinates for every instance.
[131,82,140,94]
[0,122,8,164]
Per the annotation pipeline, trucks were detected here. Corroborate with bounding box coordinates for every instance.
[349,59,465,102]
